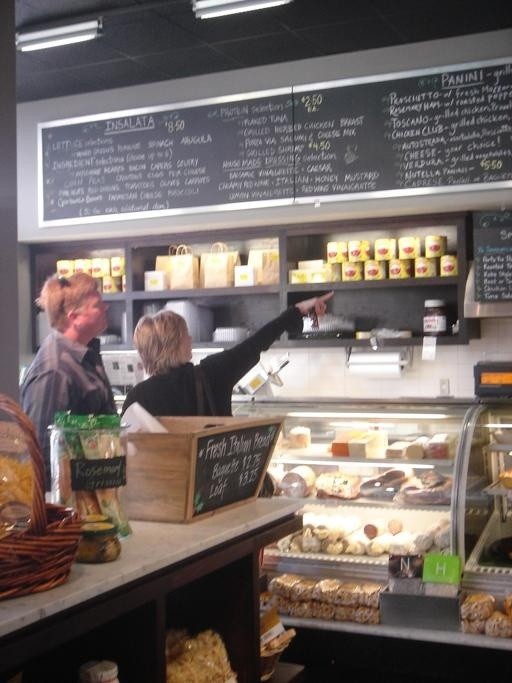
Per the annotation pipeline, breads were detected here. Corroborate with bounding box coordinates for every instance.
[288,426,312,449]
[392,470,452,506]
[387,439,407,459]
[349,429,387,457]
[277,466,316,497]
[360,470,406,503]
[316,472,359,500]
[429,431,456,458]
[332,428,365,456]
[406,432,429,460]
[497,468,512,491]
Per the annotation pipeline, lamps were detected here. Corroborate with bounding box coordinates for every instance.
[15,14,109,54]
[192,0,289,19]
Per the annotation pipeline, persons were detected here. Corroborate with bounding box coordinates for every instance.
[16,270,120,497]
[122,292,339,419]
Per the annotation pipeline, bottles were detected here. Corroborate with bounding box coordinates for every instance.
[72,660,120,683]
[421,299,448,336]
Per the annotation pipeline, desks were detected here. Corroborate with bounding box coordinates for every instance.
[0,493,311,682]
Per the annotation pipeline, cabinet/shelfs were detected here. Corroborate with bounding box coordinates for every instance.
[27,219,465,347]
[257,407,512,649]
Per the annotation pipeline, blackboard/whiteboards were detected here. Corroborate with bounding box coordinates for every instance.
[191,422,283,519]
[37,56,512,230]
[474,209,512,302]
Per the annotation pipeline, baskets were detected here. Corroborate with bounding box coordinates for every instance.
[0,394,85,600]
